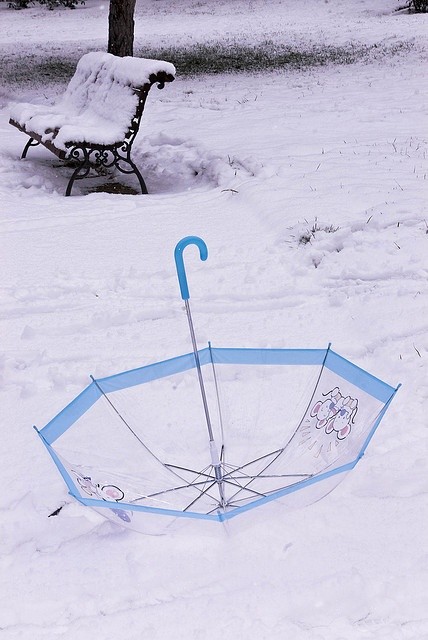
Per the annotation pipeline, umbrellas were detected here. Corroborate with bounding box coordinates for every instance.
[33,234,402,540]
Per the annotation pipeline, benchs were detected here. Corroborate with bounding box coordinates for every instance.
[7,49,176,197]
